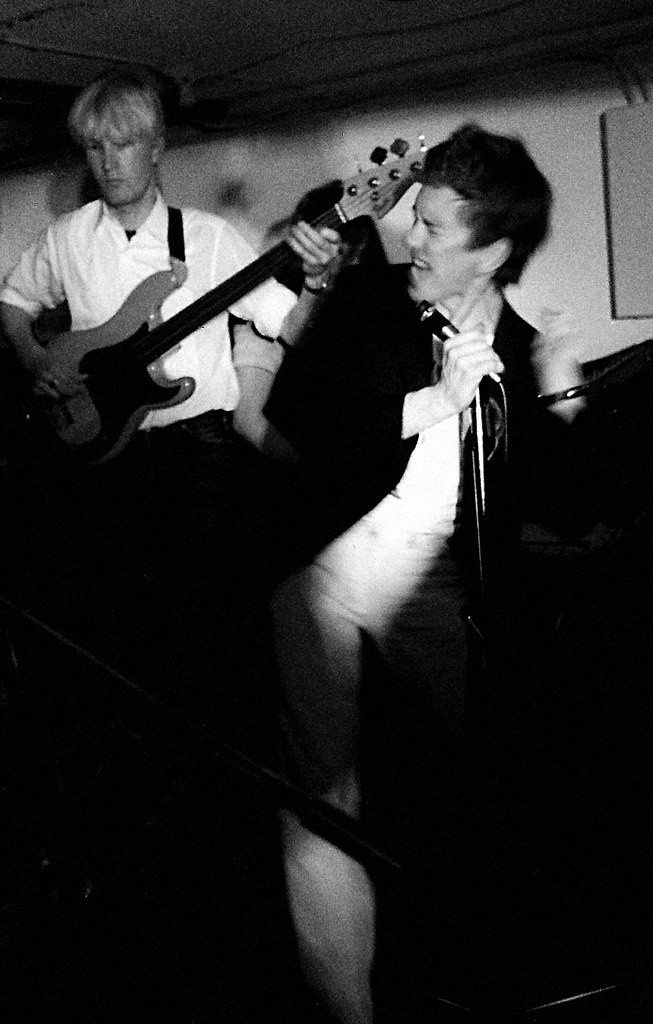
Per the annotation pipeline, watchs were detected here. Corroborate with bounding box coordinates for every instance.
[304,271,335,294]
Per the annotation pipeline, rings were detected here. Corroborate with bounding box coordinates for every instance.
[52,379,61,387]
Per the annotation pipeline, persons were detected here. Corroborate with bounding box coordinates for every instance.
[0,73,341,756]
[257,125,646,1024]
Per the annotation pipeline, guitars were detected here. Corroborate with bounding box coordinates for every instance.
[26,131,428,472]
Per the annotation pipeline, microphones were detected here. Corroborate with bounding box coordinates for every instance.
[414,299,502,383]
